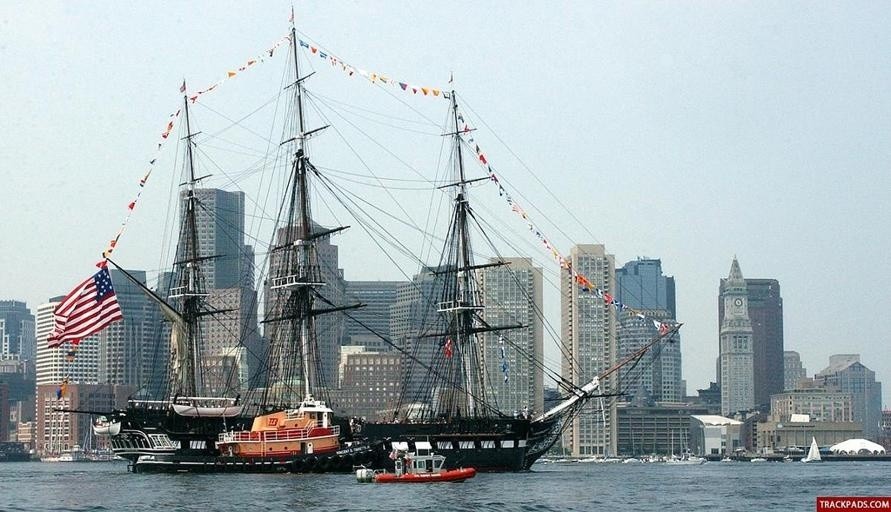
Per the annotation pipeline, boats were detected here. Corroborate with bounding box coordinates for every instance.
[40,443,122,462]
[535,447,707,465]
[801,436,823,464]
[751,457,767,463]
[373,454,475,483]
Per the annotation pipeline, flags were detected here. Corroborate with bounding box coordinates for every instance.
[56,380,68,400]
[300,41,450,99]
[445,339,452,357]
[66,339,80,359]
[498,338,509,383]
[180,85,186,92]
[47,268,124,348]
[190,36,291,103]
[96,109,181,268]
[458,113,669,335]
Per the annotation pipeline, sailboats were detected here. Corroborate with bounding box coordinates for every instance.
[50,1,684,473]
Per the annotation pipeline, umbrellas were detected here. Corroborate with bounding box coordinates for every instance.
[819,448,831,454]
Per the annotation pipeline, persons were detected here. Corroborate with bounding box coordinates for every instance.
[406,458,411,473]
[426,461,430,470]
[230,423,242,431]
